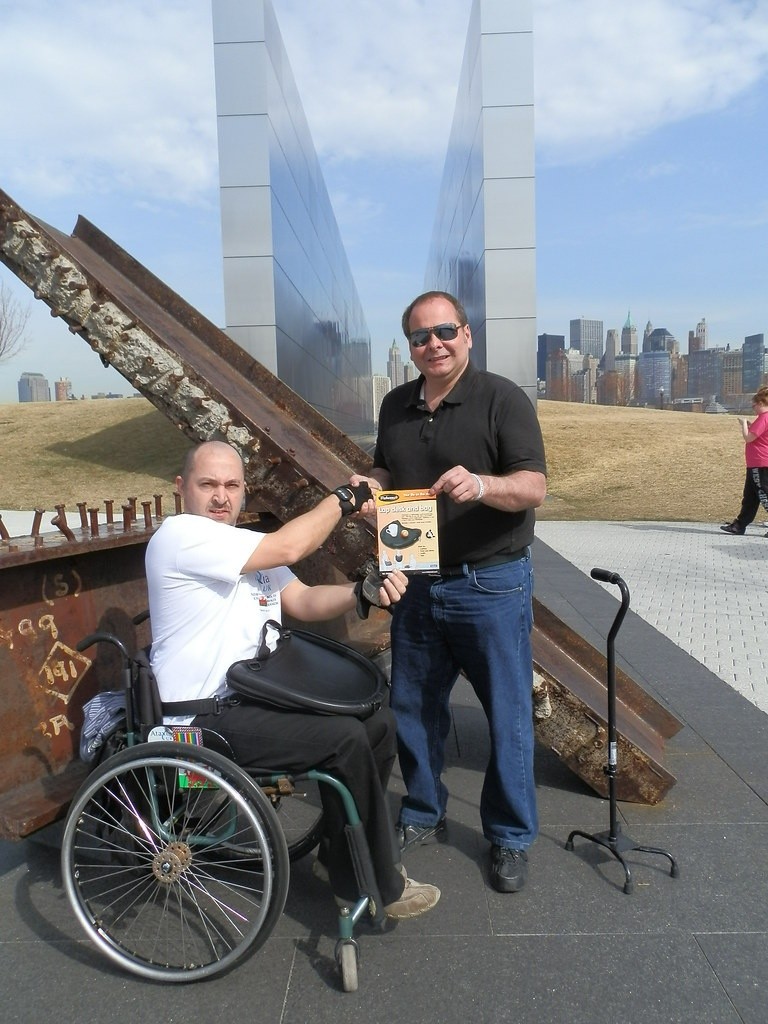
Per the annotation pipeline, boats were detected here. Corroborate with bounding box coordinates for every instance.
[705,402,729,413]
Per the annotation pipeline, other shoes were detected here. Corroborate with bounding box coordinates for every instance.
[765,532,768,538]
[720,518,745,535]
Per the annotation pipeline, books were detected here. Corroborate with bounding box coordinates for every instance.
[376,489,441,577]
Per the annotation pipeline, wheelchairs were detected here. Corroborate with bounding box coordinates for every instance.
[59,611,397,993]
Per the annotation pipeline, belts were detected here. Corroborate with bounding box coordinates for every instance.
[429,546,528,578]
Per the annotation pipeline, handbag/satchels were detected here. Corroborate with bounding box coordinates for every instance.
[227,620,390,719]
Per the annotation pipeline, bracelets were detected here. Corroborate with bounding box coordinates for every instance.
[471,474,485,502]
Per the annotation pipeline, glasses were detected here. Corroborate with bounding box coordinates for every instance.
[408,323,462,346]
[751,403,759,409]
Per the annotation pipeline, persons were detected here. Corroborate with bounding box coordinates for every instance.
[348,291,547,893]
[144,440,441,919]
[720,387,768,538]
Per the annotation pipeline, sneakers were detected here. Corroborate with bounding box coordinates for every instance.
[312,855,407,886]
[490,843,528,892]
[395,817,447,856]
[335,878,440,920]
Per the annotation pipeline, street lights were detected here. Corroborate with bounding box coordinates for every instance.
[659,386,664,410]
